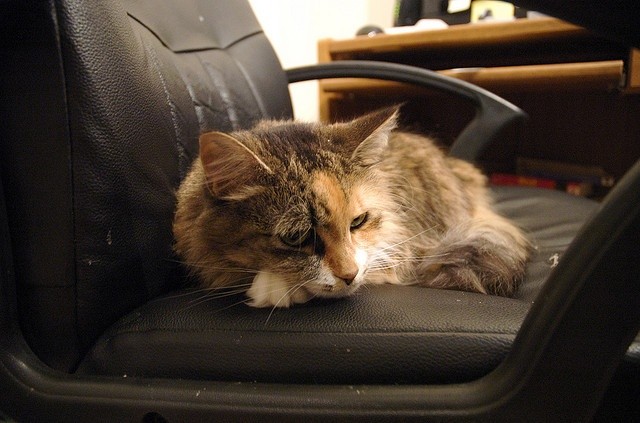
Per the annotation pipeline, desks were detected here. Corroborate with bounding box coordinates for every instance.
[319,14,638,189]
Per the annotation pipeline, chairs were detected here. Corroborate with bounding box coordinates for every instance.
[0,0,639,420]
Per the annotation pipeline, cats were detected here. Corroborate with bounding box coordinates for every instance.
[172,101,534,327]
